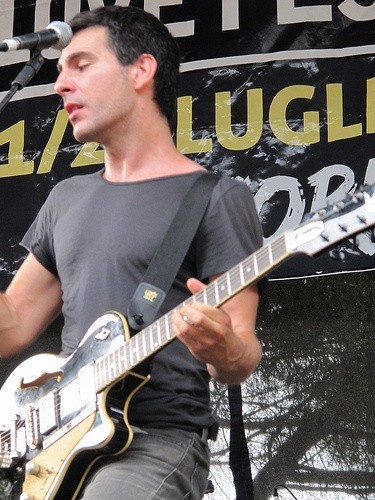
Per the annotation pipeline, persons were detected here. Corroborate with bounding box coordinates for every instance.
[1,6,261,500]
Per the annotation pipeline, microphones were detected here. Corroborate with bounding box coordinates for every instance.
[0,20,74,51]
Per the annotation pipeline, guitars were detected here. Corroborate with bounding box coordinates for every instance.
[0,182,375,500]
